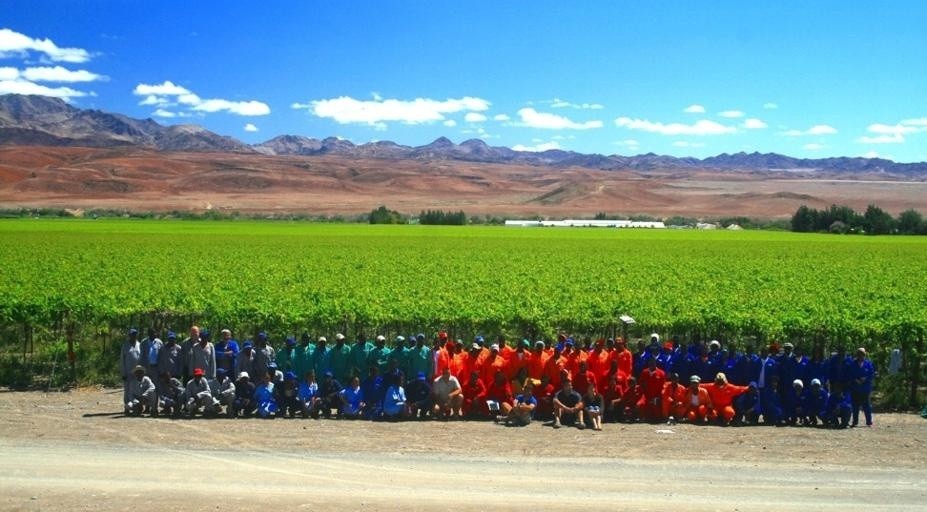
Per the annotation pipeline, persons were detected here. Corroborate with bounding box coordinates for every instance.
[120,327,874,430]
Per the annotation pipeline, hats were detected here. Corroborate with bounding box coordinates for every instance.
[131,362,427,387]
[128,326,267,349]
[668,371,849,397]
[637,333,867,355]
[469,334,624,354]
[285,331,464,350]
